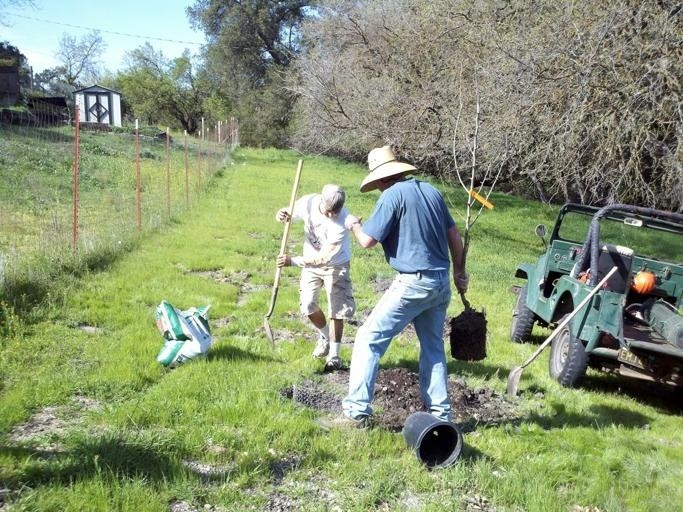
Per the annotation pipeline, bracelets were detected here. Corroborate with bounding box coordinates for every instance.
[349,220,359,230]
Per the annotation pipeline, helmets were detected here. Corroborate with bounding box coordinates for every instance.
[631,271,655,295]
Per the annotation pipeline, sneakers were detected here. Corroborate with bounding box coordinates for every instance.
[324,355,341,371]
[312,339,329,357]
[314,413,367,431]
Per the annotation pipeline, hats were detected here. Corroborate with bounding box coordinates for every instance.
[359,145,417,193]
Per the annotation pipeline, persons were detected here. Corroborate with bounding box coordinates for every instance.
[276,184,355,368]
[319,147,469,435]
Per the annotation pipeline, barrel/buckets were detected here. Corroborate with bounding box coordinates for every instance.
[403,411,462,469]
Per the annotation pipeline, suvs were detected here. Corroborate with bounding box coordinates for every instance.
[510,203,681,387]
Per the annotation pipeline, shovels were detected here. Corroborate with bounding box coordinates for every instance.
[263,159,303,350]
[506,266,619,397]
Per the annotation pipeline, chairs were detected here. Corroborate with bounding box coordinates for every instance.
[599,242,635,293]
